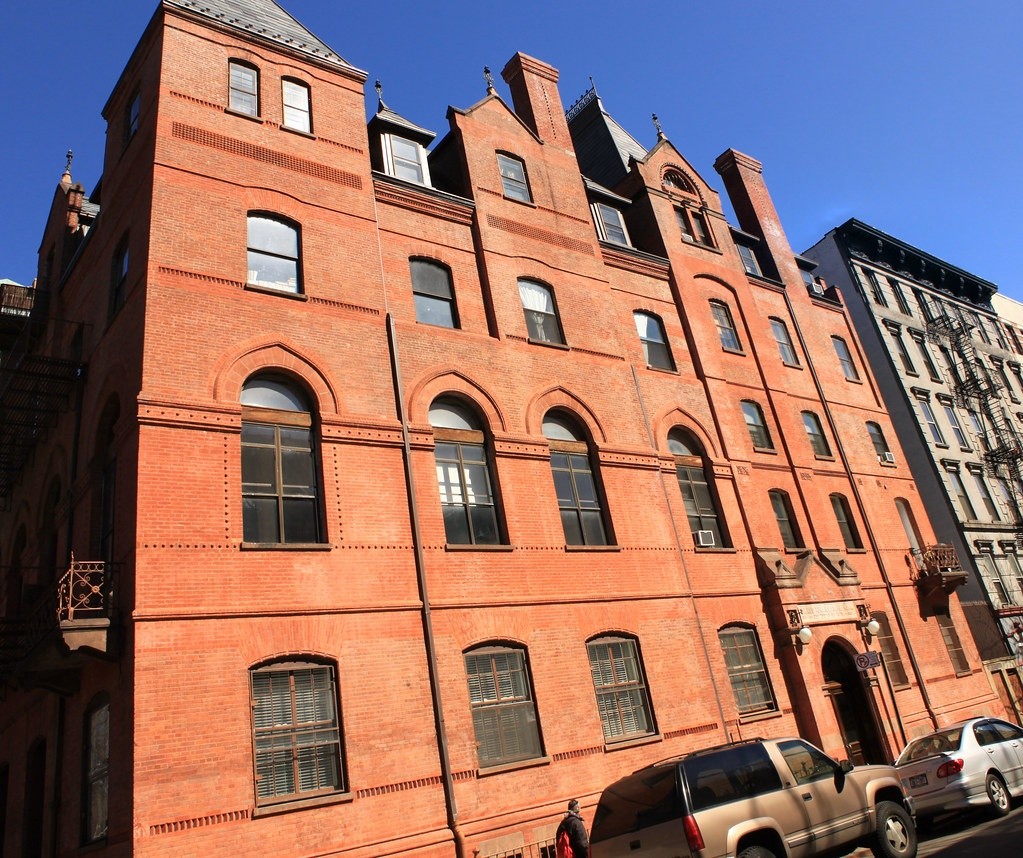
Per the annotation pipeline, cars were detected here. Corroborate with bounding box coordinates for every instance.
[893,714,1023,832]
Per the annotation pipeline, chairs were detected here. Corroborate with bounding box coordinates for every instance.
[696,785,717,807]
[752,767,781,792]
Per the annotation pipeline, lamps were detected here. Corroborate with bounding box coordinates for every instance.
[858,603,880,635]
[789,609,812,643]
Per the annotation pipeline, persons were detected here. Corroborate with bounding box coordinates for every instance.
[556,800,589,858]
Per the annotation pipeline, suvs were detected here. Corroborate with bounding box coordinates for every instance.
[586,735,918,858]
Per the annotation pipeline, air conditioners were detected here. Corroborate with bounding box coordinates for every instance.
[682,233,693,242]
[882,452,896,463]
[691,530,715,546]
[808,283,824,297]
